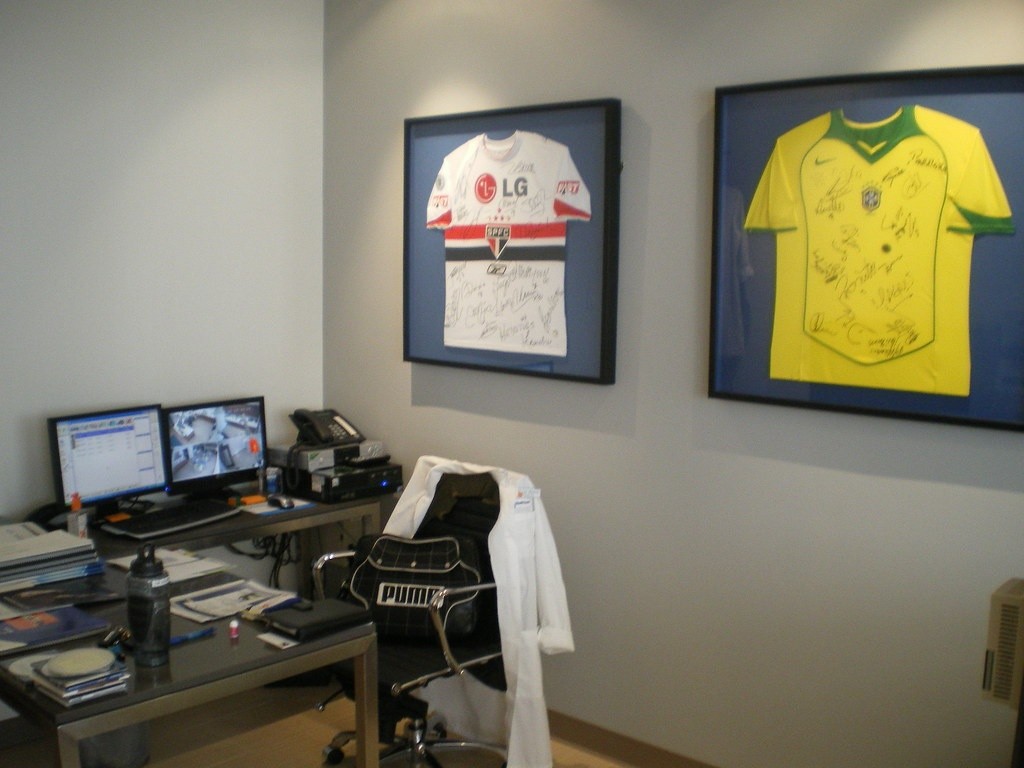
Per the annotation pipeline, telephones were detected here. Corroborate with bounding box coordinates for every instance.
[287,407,367,450]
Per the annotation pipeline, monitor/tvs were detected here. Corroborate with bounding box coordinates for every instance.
[46,395,269,531]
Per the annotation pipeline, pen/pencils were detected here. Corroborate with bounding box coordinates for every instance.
[171,627,218,646]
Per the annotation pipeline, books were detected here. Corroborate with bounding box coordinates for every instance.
[262,598,372,642]
[0,521,131,707]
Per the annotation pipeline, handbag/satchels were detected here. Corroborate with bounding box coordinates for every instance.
[342,530,482,656]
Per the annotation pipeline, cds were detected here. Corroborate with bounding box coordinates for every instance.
[41,647,116,677]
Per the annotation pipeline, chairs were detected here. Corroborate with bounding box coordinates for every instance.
[311,455,538,768]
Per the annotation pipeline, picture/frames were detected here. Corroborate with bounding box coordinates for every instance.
[402,97,621,385]
[709,63,1024,433]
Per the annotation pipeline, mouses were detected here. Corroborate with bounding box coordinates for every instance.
[267,493,295,509]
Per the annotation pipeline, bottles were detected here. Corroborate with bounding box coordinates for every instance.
[126,543,172,667]
[67,493,87,540]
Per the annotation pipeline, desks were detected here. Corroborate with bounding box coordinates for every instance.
[0,492,382,768]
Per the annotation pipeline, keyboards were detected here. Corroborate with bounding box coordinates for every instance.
[101,499,241,542]
